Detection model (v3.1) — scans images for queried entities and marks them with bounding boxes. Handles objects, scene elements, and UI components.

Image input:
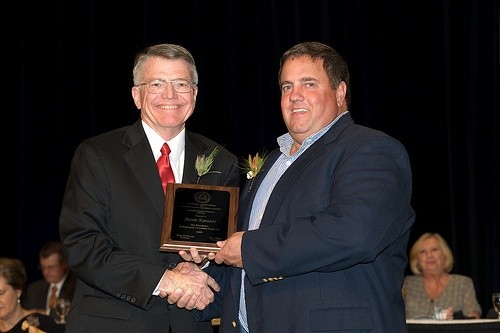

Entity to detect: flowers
[232,151,272,193]
[194,145,223,184]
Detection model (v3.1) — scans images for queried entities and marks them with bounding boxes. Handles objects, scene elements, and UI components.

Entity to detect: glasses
[135,78,196,93]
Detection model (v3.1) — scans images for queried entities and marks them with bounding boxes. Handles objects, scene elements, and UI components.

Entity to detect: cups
[433,298,450,321]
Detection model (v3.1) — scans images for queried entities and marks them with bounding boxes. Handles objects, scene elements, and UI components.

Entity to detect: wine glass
[55,299,70,324]
[492,294,500,321]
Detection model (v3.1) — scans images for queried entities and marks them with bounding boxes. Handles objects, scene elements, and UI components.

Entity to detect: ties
[49,286,57,309]
[156,143,176,196]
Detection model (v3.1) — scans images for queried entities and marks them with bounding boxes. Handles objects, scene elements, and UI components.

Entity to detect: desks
[406,317,500,333]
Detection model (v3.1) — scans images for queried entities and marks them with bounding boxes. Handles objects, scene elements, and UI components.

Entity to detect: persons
[58,43,243,333]
[0,258,61,333]
[157,41,416,333]
[22,241,77,333]
[401,232,482,320]
